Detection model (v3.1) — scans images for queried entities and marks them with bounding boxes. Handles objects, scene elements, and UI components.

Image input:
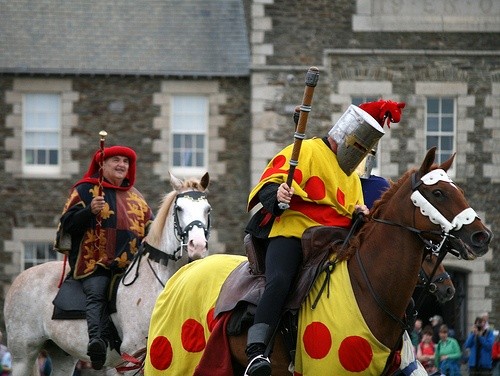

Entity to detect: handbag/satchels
[439,359,462,376]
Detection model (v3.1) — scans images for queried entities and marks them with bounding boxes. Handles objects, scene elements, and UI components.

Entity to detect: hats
[429,315,443,326]
[475,313,488,326]
[73,145,136,191]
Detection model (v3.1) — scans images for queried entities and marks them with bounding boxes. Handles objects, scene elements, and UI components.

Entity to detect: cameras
[474,317,483,330]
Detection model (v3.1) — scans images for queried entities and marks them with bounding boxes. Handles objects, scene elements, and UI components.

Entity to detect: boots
[81,276,110,371]
[245,323,273,376]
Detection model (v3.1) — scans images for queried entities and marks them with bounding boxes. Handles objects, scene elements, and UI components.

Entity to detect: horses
[143,146,493,376]
[4,171,212,376]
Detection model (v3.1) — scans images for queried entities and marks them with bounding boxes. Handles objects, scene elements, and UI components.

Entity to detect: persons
[244,99,405,376]
[409,310,500,376]
[0,330,51,376]
[53,135,155,371]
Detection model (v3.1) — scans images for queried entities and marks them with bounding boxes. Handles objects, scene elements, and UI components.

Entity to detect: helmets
[328,104,386,180]
